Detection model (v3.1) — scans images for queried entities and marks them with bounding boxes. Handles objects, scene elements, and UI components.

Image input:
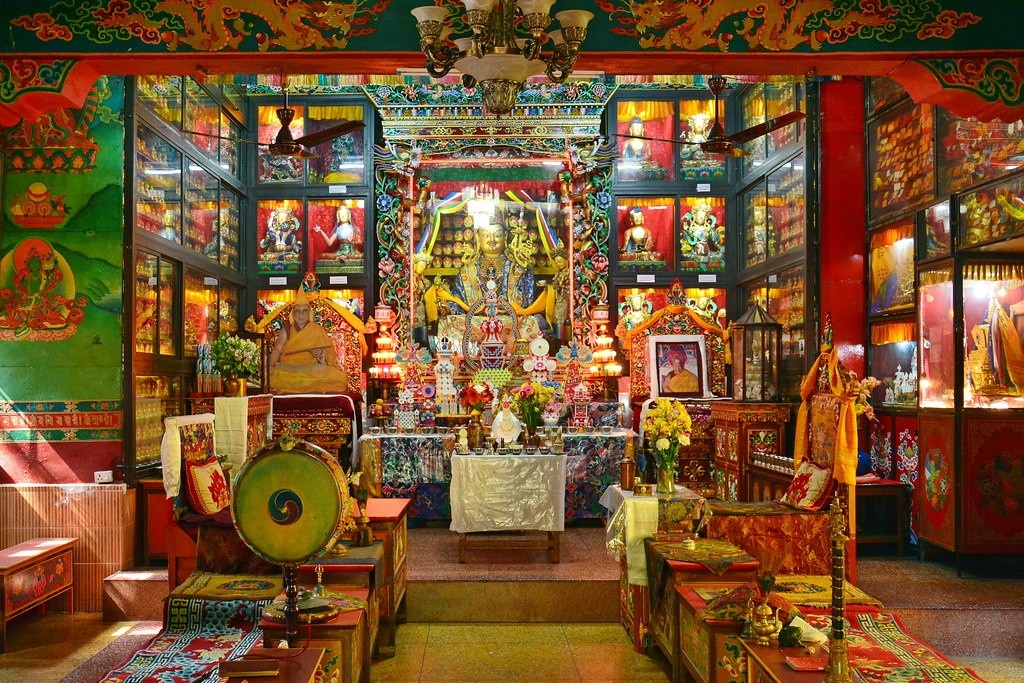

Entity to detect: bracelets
[320,353,325,357]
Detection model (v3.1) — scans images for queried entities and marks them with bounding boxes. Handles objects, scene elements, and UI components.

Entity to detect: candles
[369,325,401,378]
[589,325,622,376]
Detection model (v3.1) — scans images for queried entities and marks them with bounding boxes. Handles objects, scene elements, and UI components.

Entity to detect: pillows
[774,454,835,513]
[184,455,231,515]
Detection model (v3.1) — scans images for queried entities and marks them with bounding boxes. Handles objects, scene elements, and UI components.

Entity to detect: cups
[473,448,484,454]
[539,447,550,454]
[524,448,537,454]
[454,447,459,453]
[497,448,509,454]
[511,448,522,455]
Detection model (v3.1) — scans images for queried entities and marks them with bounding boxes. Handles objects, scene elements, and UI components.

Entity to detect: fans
[611,75,808,158]
[180,72,366,158]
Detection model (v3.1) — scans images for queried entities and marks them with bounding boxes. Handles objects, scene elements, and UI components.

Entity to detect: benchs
[856,476,910,556]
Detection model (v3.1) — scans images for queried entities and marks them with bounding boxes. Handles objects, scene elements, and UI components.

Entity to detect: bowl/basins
[633,483,652,495]
[368,427,468,435]
[535,426,615,434]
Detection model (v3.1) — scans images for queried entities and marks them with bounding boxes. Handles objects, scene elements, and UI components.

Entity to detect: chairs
[703,313,860,585]
[160,412,283,577]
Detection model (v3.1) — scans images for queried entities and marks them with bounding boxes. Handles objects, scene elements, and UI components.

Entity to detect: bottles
[683,536,695,551]
[621,456,635,489]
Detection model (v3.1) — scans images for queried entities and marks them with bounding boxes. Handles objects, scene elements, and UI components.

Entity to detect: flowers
[458,380,555,428]
[212,332,262,378]
[644,396,692,492]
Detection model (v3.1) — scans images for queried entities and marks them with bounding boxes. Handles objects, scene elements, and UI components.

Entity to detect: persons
[662,344,698,392]
[260,206,304,253]
[618,205,662,261]
[680,111,713,161]
[314,204,365,259]
[436,202,556,357]
[681,200,723,261]
[618,116,661,168]
[269,280,348,393]
[619,292,650,330]
[685,288,717,317]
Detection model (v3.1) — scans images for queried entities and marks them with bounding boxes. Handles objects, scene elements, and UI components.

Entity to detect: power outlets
[94,470,113,483]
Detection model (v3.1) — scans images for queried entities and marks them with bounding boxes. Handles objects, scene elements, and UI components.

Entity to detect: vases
[467,416,540,450]
[222,374,240,397]
[656,462,675,493]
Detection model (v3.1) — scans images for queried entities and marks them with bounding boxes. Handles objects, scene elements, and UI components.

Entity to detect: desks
[709,401,793,503]
[359,428,639,519]
[450,447,567,562]
[599,484,865,683]
[272,394,365,497]
[139,464,233,566]
[0,537,79,654]
[631,395,732,488]
[175,498,413,683]
[214,394,274,489]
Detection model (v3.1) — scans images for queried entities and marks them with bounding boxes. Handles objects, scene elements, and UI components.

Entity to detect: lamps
[411,0,594,120]
